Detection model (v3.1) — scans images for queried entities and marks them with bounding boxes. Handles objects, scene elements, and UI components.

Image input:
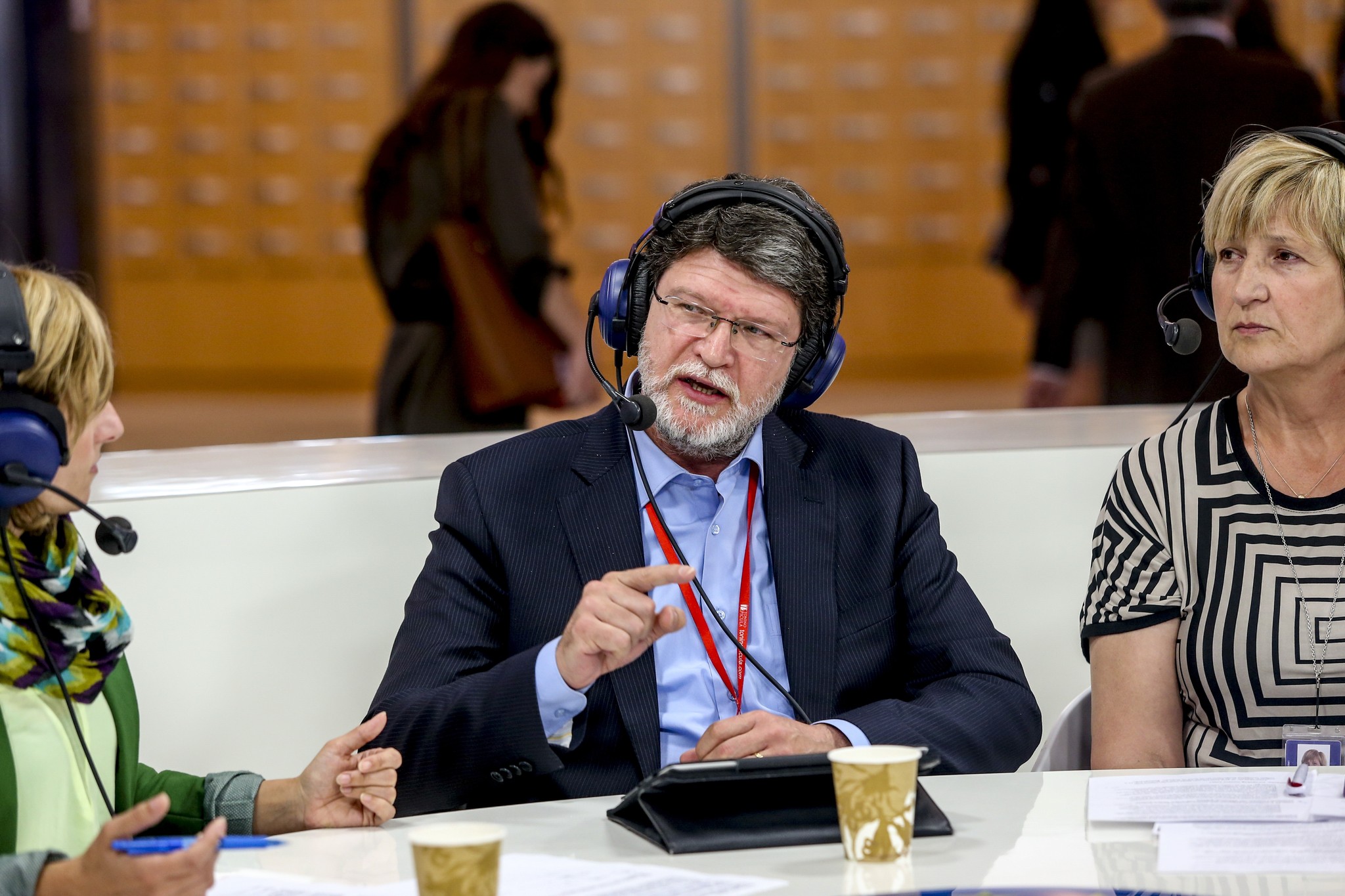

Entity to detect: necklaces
[1244,392,1345,499]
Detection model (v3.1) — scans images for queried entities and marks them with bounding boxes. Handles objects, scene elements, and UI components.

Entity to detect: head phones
[0,269,66,505]
[593,181,849,409]
[1190,126,1344,321]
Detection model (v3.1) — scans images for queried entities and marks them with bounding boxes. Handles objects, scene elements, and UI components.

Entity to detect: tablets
[615,742,941,818]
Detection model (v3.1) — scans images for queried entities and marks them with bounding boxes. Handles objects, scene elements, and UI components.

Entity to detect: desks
[203,762,1345,894]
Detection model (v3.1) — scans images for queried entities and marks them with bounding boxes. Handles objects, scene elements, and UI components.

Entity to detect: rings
[752,753,765,759]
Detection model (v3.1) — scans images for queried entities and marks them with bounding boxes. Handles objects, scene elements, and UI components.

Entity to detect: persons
[358,0,601,437]
[0,258,405,896]
[1301,750,1327,767]
[986,0,1118,409]
[353,169,1043,821]
[1076,126,1345,770]
[1021,1,1327,417]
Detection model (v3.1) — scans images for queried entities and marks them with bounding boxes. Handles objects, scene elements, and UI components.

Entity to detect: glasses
[653,281,810,362]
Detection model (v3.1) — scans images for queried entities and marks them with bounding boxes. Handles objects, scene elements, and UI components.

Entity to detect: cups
[828,745,922,863]
[412,821,506,895]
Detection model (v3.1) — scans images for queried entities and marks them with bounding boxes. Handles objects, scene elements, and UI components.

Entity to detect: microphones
[5,462,137,554]
[1156,279,1201,355]
[583,294,657,430]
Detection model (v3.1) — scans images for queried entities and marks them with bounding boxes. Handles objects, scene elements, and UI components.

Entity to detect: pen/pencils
[1286,764,1308,795]
[115,836,285,857]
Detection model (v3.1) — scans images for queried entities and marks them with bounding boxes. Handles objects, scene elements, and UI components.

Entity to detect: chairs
[1031,684,1093,772]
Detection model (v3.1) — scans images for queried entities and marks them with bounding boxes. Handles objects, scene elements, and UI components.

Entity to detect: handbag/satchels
[426,220,572,409]
